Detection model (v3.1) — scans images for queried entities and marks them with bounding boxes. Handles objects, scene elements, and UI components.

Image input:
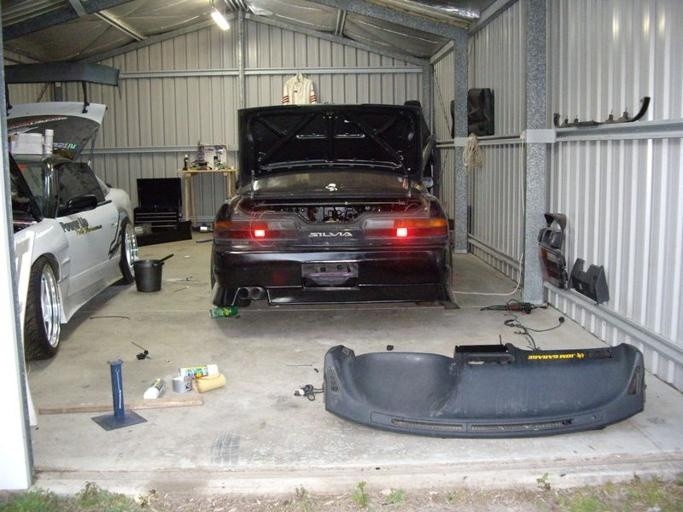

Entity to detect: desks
[180,168,237,221]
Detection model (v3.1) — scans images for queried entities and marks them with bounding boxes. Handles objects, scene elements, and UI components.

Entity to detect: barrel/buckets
[133,259,164,292]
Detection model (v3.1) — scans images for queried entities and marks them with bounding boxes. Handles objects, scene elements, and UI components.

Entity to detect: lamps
[209,0,231,32]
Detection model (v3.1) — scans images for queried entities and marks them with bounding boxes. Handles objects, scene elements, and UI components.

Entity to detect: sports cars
[209,102,454,307]
[8,101,140,362]
